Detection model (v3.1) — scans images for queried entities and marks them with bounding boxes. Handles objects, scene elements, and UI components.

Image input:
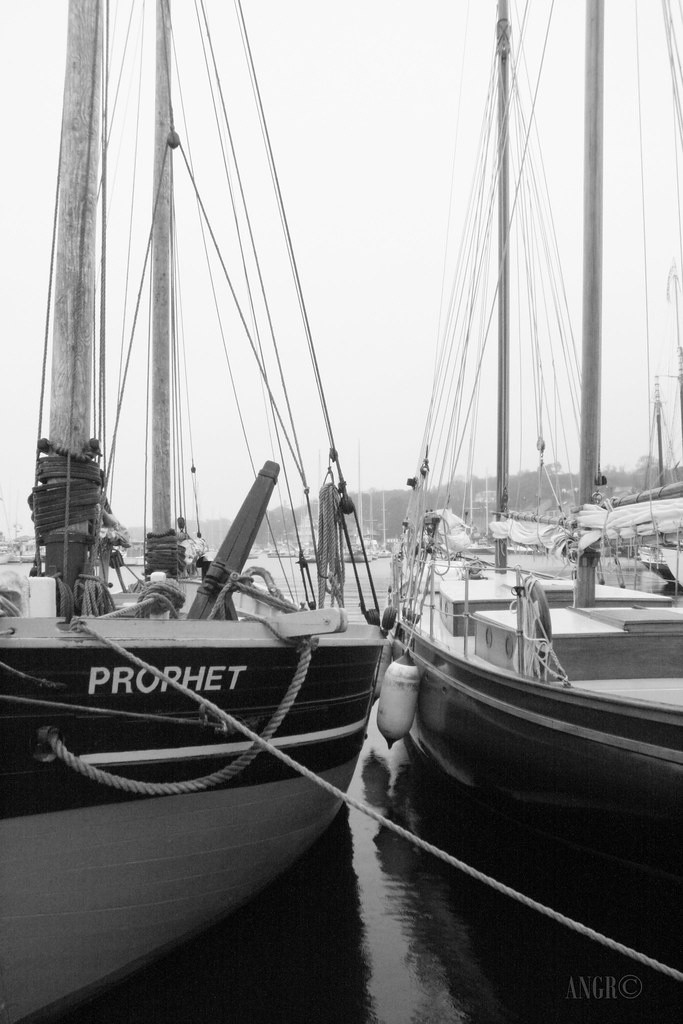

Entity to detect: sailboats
[1,426,681,573]
[376,1,682,885]
[1,0,403,1024]
